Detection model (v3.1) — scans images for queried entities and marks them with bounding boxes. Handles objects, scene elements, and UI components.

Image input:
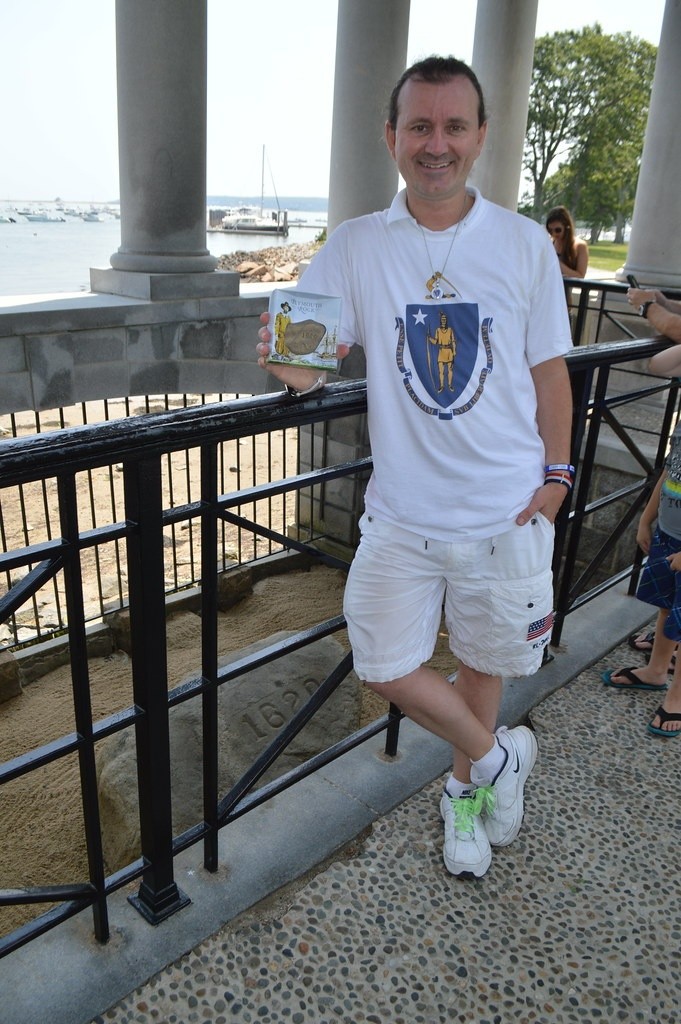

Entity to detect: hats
[281,301,291,311]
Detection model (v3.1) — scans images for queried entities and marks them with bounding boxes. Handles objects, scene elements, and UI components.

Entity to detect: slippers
[602,666,667,690]
[646,704,681,737]
[628,631,678,675]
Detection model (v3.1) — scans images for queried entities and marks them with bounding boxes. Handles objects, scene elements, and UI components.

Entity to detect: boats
[206,143,328,237]
[0,191,120,226]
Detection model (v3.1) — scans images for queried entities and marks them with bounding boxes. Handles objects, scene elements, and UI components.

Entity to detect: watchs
[638,300,656,319]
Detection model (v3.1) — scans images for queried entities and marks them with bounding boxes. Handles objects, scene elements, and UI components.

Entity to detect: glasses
[547,226,569,233]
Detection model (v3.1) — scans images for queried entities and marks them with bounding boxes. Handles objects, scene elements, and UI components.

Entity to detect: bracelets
[544,463,575,473]
[544,473,573,489]
[285,368,327,399]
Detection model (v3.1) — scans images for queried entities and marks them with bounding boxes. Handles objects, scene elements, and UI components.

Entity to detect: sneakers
[469,726,538,848]
[439,785,491,878]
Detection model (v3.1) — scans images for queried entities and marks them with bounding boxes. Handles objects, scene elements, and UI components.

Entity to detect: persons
[545,206,589,340]
[273,302,292,361]
[628,340,681,676]
[256,59,580,882]
[601,411,681,738]
[624,273,681,345]
[428,311,457,393]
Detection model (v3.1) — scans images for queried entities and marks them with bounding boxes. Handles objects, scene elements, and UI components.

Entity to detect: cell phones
[627,274,639,288]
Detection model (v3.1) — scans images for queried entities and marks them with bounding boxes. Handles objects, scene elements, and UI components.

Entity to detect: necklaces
[407,191,467,300]
